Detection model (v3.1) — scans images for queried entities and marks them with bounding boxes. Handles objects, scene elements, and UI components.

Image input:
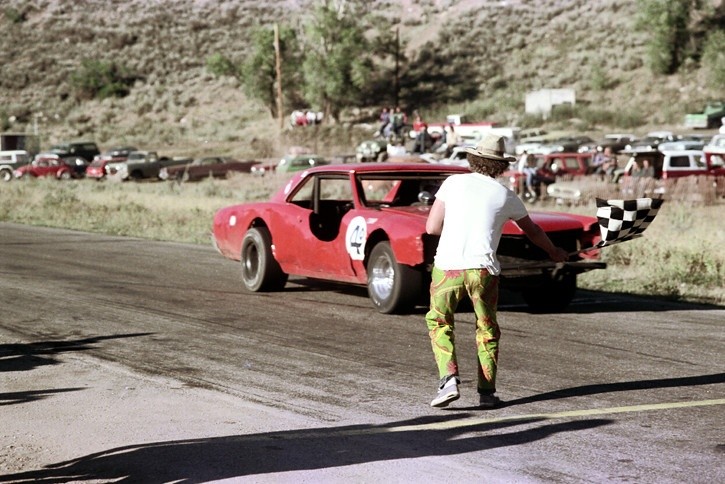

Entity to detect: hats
[464,132,516,162]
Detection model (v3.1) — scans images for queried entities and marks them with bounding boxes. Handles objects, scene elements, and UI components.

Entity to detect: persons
[519,143,616,201]
[425,133,568,406]
[291,107,437,153]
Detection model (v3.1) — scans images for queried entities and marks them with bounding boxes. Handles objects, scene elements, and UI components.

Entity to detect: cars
[275,152,331,174]
[157,154,262,184]
[249,159,281,176]
[0,141,195,181]
[419,111,725,209]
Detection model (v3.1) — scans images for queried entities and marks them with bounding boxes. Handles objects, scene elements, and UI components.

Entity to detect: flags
[595,197,664,249]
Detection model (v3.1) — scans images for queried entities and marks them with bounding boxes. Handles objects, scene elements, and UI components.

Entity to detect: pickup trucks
[682,101,725,129]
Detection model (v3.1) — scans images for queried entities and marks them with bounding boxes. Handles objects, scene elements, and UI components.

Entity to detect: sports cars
[211,162,609,316]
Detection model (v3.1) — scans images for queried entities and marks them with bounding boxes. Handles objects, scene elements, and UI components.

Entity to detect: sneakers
[430,384,460,407]
[480,395,500,406]
